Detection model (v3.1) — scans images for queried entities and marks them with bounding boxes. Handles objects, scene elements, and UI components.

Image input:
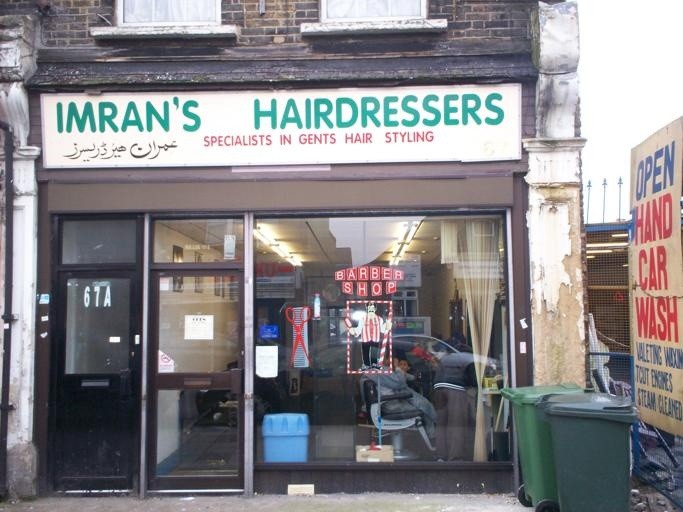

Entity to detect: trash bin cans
[499,381,638,512]
[262,412,310,463]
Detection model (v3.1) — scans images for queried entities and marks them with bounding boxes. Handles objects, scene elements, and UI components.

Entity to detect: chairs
[362,375,438,452]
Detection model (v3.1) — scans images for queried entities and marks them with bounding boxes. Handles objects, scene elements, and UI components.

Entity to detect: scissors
[285,306,312,368]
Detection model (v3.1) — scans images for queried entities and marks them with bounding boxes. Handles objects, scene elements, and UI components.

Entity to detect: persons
[371,348,438,440]
[349,301,392,371]
[432,341,502,463]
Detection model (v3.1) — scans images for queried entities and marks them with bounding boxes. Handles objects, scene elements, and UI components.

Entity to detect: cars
[280,334,498,420]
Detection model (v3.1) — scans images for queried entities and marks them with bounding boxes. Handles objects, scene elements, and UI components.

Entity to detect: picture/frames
[171,245,227,297]
